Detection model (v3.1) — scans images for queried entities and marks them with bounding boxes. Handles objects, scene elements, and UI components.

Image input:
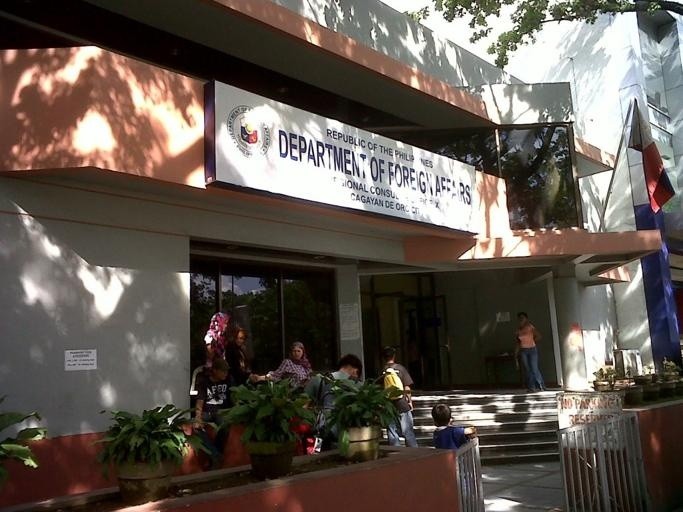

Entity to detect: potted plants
[315,370,412,462]
[218,375,316,478]
[90,403,218,503]
[592,356,683,406]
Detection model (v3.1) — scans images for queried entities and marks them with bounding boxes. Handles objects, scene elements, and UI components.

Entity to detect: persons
[190,311,362,455]
[432,404,477,451]
[513,312,546,394]
[376,346,420,449]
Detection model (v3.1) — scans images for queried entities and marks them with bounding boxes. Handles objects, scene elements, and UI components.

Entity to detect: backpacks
[381,364,403,400]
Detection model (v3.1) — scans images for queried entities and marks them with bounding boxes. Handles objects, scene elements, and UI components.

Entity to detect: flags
[626,99,676,217]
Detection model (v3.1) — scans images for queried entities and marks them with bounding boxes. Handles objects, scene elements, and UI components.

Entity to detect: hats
[214,358,230,370]
